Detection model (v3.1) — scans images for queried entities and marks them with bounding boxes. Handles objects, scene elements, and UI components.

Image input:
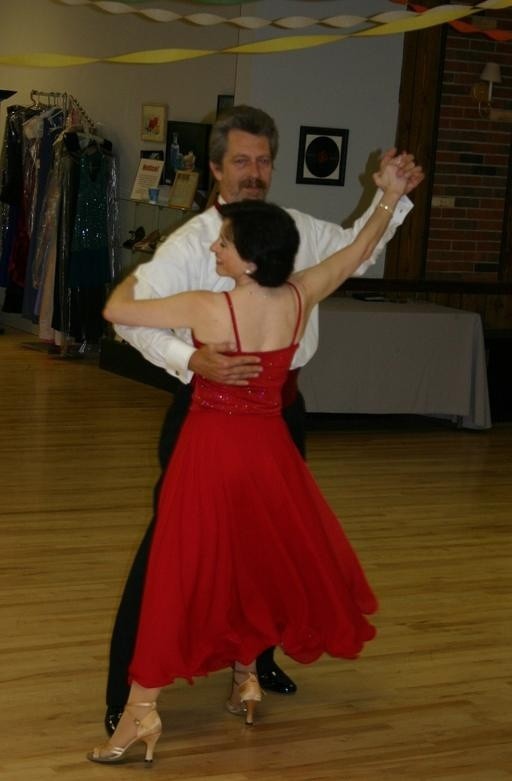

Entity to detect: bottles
[174,152,183,173]
[170,131,179,168]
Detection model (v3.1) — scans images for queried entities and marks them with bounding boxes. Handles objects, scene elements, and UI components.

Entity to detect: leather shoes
[257,657,297,695]
[104,701,126,737]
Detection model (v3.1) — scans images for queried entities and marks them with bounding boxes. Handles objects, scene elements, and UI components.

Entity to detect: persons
[103,103,427,740]
[86,155,414,766]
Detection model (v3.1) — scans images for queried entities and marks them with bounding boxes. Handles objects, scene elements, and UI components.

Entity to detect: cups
[148,187,160,205]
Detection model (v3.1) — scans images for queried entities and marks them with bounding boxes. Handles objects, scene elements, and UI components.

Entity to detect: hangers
[11,89,103,147]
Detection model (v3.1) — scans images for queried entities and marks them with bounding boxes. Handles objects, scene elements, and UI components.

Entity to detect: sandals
[122,225,170,253]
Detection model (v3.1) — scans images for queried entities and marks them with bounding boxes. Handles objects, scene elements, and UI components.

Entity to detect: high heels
[224,670,262,727]
[85,700,163,764]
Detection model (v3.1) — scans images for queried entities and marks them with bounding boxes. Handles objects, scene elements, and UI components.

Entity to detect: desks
[295,296,494,432]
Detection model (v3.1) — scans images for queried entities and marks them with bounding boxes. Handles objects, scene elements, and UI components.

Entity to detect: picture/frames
[216,93,235,120]
[295,125,349,187]
[166,169,200,209]
[141,103,169,144]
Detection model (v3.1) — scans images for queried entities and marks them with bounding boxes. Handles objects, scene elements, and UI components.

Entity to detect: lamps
[470,61,502,119]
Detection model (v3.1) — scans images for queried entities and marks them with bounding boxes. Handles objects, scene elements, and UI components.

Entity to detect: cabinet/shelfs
[118,198,202,255]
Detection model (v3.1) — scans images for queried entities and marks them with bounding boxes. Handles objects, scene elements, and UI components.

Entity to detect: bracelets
[376,202,394,216]
